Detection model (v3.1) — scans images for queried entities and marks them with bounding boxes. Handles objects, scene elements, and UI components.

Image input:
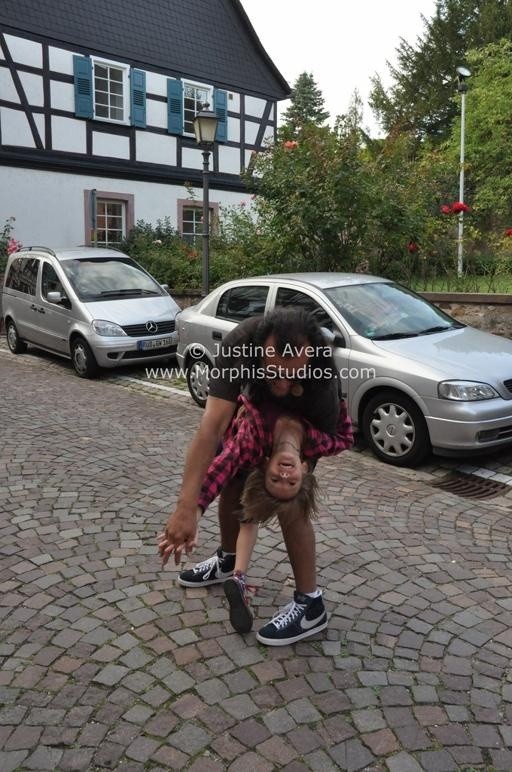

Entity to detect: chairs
[224,294,253,321]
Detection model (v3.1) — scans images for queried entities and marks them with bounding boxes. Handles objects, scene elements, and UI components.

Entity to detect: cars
[173,271,512,465]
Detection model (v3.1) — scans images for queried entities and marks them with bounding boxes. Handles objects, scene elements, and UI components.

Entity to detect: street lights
[192,101,222,299]
[453,65,472,279]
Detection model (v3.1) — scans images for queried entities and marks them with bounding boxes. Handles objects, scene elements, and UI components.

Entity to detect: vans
[2,244,184,380]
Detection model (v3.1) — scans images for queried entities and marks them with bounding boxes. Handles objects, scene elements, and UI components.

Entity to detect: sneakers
[223,571,254,633]
[177,546,236,588]
[255,590,328,648]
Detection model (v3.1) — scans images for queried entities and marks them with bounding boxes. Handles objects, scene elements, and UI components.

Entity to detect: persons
[159,306,344,646]
[156,390,354,633]
